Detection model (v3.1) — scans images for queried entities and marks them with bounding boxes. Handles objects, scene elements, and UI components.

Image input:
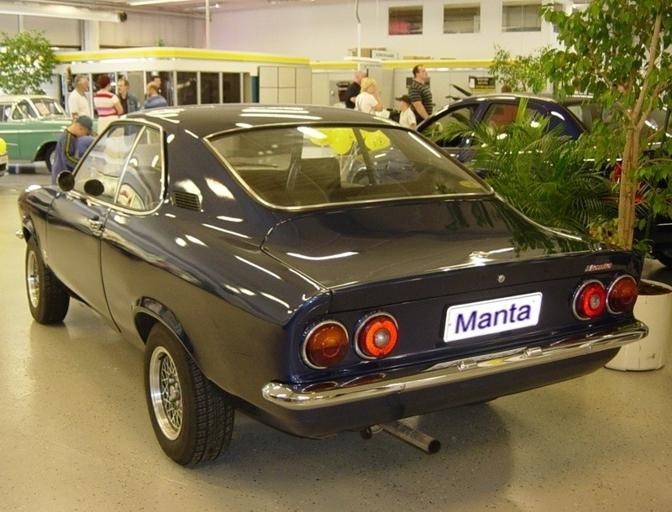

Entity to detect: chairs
[286,157,342,191]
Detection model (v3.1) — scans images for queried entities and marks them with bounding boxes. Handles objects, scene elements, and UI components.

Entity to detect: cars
[0,137,9,177]
[16,102,650,469]
[0,95,98,172]
[346,93,672,269]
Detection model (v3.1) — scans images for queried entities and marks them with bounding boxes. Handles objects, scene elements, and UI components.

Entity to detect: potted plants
[488,0,672,372]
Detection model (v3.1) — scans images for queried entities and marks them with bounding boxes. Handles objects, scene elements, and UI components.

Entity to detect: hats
[78,116,92,128]
[361,79,374,91]
[396,96,410,102]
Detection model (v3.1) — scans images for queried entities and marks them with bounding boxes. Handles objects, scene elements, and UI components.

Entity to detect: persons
[354,77,384,115]
[50,114,92,185]
[408,63,433,125]
[346,70,364,110]
[68,74,168,143]
[395,94,417,130]
